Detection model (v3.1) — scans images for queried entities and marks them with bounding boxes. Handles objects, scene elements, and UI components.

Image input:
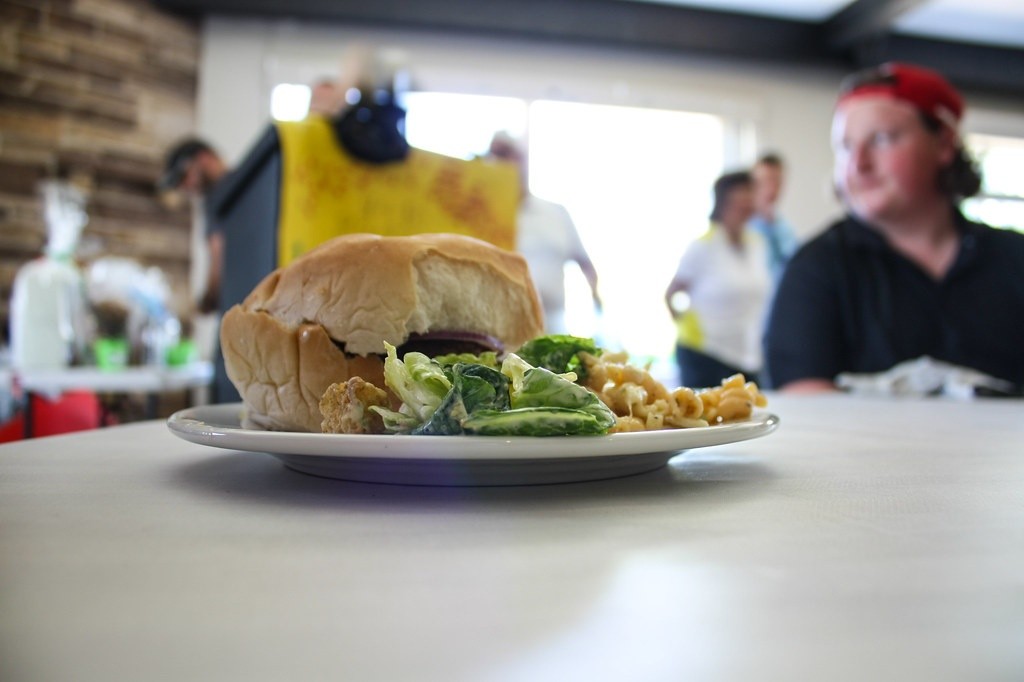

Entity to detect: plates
[166,403,779,488]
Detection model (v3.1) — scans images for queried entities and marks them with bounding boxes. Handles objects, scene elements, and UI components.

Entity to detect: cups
[94,339,129,368]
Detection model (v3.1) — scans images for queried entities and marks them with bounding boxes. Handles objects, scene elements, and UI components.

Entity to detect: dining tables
[0,397,1024,682]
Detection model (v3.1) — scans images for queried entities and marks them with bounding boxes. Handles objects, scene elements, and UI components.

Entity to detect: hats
[832,60,967,136]
[708,172,751,221]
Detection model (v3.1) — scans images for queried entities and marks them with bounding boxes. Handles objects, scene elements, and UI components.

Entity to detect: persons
[752,156,801,389]
[482,134,605,335]
[667,173,773,386]
[766,61,1024,403]
[160,134,244,403]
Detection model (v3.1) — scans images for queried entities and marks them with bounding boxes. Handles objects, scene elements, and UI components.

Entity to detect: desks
[20,366,213,418]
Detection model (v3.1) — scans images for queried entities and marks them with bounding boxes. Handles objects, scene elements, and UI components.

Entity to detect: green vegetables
[365,332,618,437]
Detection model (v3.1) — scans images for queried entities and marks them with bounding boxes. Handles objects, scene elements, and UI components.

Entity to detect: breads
[218,234,543,429]
